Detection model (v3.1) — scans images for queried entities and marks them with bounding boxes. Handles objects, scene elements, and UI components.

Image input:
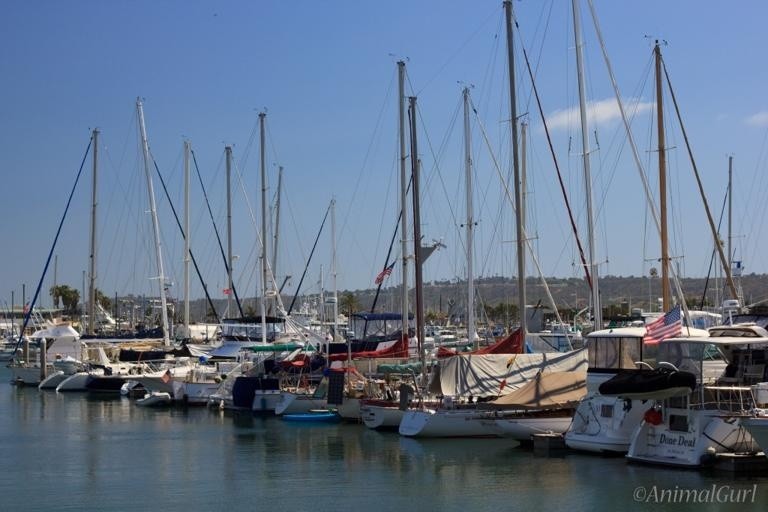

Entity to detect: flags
[375,262,395,285]
[643,303,682,345]
